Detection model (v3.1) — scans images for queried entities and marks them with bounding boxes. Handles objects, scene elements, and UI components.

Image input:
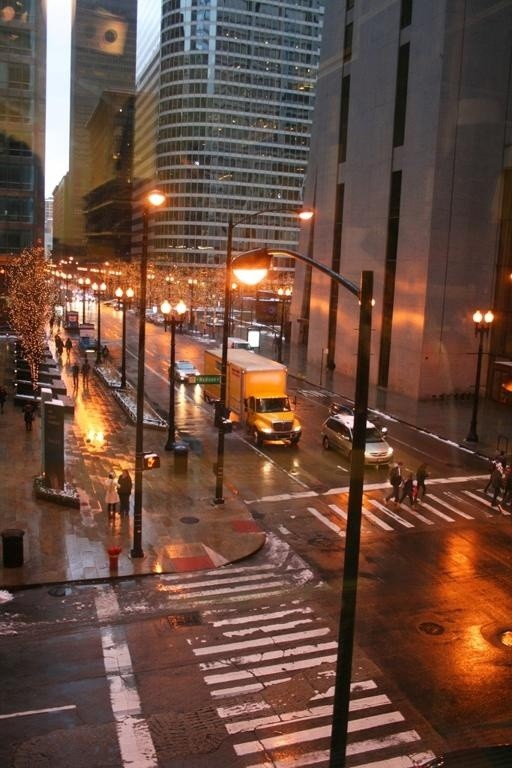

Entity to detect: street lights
[214,205,317,505]
[161,298,187,449]
[43,257,291,387]
[132,188,165,559]
[466,309,494,444]
[232,246,374,768]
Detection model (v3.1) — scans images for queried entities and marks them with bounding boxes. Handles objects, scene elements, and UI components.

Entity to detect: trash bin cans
[174,445,188,474]
[0,529,26,568]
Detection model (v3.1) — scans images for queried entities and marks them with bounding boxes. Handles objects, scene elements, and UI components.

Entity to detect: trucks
[202,347,302,447]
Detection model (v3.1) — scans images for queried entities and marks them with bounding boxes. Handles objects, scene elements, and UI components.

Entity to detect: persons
[483,448,511,507]
[80,359,91,386]
[103,472,121,519]
[117,468,133,514]
[101,344,110,364]
[65,337,73,355]
[72,362,80,388]
[1,384,9,414]
[399,470,415,505]
[413,461,429,503]
[21,404,35,432]
[382,464,401,505]
[49,315,64,357]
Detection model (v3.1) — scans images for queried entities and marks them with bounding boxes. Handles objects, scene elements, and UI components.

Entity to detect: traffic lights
[142,453,160,469]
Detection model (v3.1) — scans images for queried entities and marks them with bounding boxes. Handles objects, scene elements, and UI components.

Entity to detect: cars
[321,401,394,466]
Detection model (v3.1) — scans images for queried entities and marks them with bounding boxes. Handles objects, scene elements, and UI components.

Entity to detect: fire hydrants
[108,545,122,571]
